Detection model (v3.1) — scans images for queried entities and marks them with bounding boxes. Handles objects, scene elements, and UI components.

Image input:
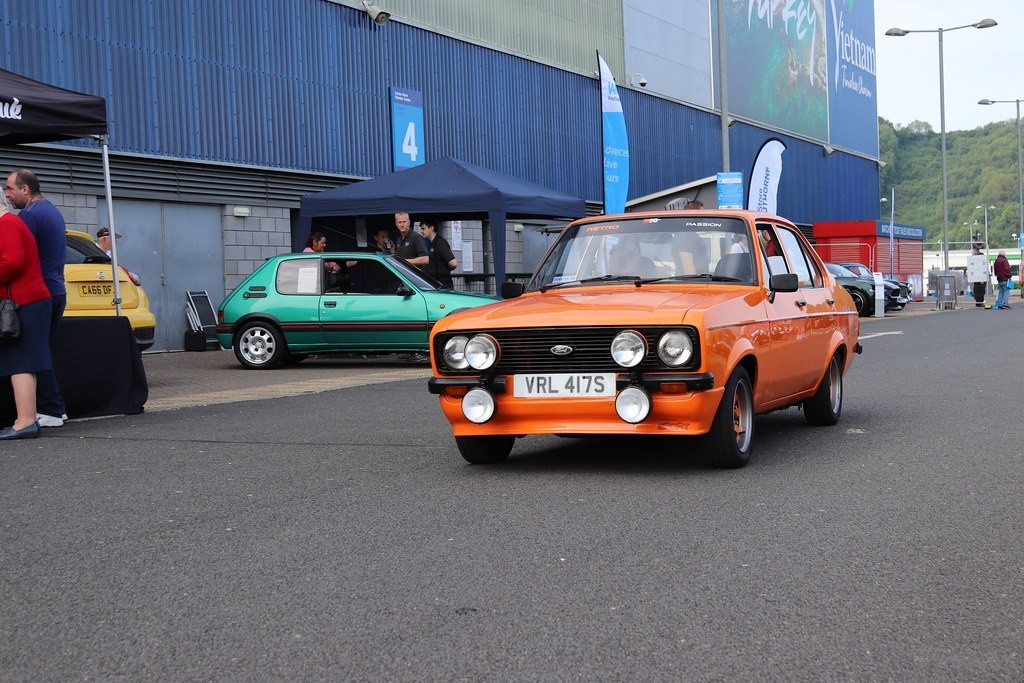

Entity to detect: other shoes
[998,305,1006,309]
[976,304,984,307]
[0,420,40,440]
[1003,304,1010,308]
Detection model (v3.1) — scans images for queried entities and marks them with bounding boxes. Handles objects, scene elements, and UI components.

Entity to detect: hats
[97,228,121,239]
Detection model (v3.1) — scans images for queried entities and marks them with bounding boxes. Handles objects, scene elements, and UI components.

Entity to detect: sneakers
[14,414,63,426]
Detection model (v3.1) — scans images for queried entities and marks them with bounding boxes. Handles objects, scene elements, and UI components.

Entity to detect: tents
[298,156,585,295]
[0,67,122,315]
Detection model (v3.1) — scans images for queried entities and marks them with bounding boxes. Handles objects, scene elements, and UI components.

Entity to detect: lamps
[880,198,888,202]
[630,73,647,88]
[361,0,390,25]
[233,208,250,216]
[514,225,524,231]
[823,145,835,156]
[718,114,735,126]
[876,160,888,168]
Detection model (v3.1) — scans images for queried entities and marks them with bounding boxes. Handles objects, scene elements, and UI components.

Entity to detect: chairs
[327,274,352,294]
[712,252,752,284]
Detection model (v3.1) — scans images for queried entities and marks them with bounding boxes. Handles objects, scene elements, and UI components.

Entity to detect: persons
[994,250,1012,309]
[670,200,709,274]
[973,253,985,307]
[731,234,749,254]
[368,230,392,253]
[5,168,68,428]
[86,227,121,263]
[0,185,53,441]
[761,229,777,257]
[388,210,430,272]
[420,219,458,290]
[303,232,340,274]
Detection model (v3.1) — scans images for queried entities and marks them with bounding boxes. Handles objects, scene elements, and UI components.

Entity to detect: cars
[990,258,1022,289]
[62,229,156,352]
[430,208,865,469]
[834,262,914,312]
[217,251,506,371]
[824,262,888,317]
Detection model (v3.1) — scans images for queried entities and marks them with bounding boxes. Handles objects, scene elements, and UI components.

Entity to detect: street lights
[976,206,996,255]
[978,98,1023,299]
[884,18,998,273]
[963,222,979,255]
